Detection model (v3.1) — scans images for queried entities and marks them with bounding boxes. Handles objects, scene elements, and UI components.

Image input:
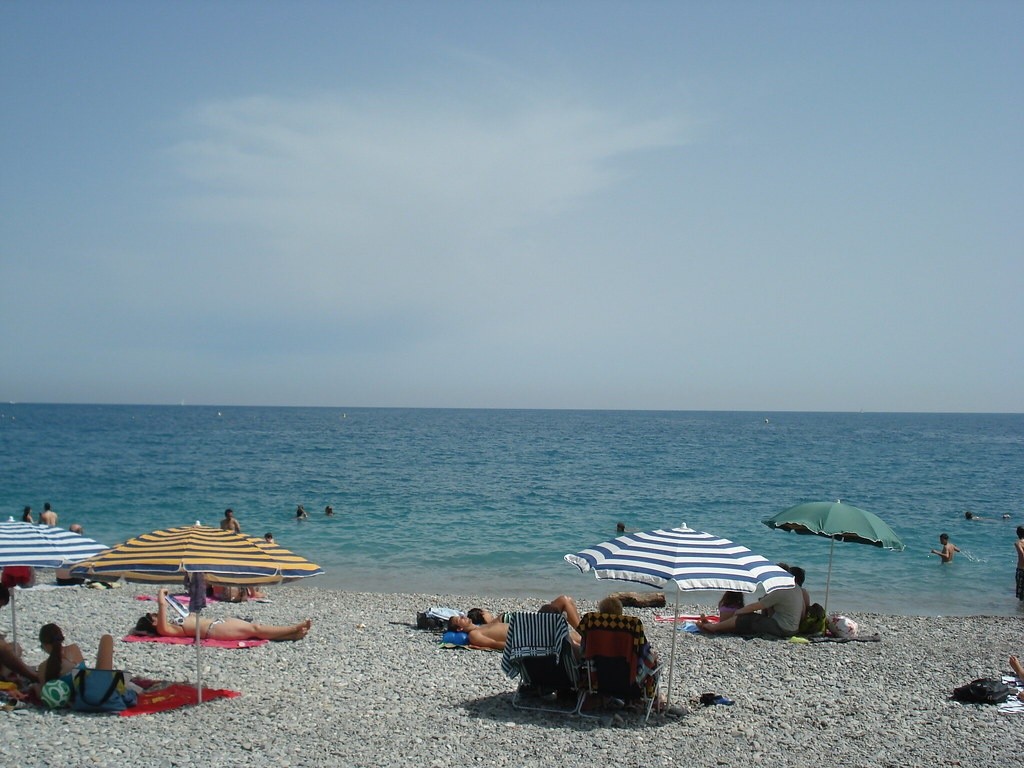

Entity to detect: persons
[22,507,34,522]
[931,534,961,564]
[615,523,628,533]
[324,506,334,517]
[69,524,82,534]
[1009,655,1024,702]
[264,533,274,543]
[1014,526,1024,601]
[296,506,307,520]
[965,512,1010,523]
[0,583,39,684]
[37,623,113,693]
[448,596,581,649]
[696,564,810,638]
[39,504,57,526]
[220,509,240,533]
[598,597,666,706]
[136,589,312,641]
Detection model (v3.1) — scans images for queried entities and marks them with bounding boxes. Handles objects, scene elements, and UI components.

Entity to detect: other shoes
[700,693,735,706]
[696,621,714,634]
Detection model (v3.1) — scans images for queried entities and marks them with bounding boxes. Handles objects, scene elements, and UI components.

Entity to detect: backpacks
[953,679,1009,704]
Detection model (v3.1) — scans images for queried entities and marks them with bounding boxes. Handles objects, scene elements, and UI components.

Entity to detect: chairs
[577,611,665,724]
[500,610,584,715]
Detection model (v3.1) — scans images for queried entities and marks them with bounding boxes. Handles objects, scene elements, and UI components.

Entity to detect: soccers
[41,679,70,709]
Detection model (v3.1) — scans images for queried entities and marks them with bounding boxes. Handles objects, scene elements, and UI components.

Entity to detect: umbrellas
[70,521,325,705]
[562,522,795,715]
[0,517,110,654]
[762,499,906,611]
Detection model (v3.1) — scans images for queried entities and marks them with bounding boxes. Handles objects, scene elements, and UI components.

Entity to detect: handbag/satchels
[799,603,858,638]
[71,667,132,711]
